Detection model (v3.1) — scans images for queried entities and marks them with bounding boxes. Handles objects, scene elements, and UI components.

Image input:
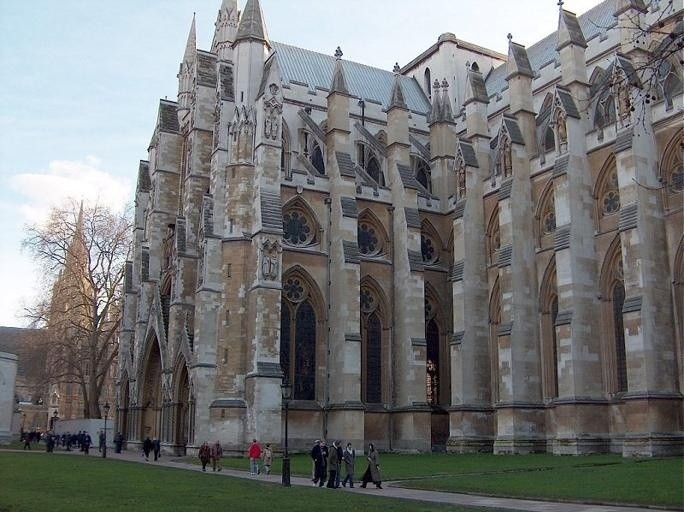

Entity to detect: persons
[311,438,384,489]
[261,442,273,474]
[211,440,223,472]
[21,430,123,456]
[143,437,151,462]
[152,439,161,461]
[197,440,210,471]
[248,438,261,475]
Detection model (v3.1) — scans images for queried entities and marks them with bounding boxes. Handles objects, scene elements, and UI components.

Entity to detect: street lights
[53,409,59,436]
[279,378,294,486]
[102,403,110,458]
